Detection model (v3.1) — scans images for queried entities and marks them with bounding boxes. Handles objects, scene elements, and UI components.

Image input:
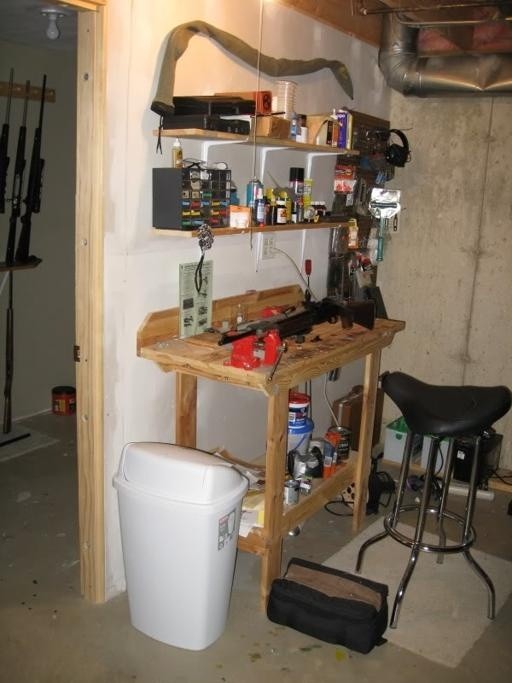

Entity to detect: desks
[137,285,405,612]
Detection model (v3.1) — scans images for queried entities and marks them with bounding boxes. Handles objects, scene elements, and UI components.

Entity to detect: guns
[217,295,376,347]
[0,67,47,267]
[2,270,13,436]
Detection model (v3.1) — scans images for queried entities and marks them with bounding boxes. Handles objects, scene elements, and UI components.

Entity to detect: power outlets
[261,234,276,259]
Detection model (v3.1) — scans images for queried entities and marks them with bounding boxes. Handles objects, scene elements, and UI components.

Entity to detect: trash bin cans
[112,441,249,651]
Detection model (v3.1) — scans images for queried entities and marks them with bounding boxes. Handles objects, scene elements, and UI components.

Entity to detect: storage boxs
[384,416,449,474]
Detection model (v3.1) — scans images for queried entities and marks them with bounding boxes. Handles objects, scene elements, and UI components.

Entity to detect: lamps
[41,9,67,40]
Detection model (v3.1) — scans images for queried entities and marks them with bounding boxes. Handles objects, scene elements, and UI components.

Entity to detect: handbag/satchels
[268,557,388,654]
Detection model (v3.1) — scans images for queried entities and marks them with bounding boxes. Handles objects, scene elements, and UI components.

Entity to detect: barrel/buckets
[287,421,314,456]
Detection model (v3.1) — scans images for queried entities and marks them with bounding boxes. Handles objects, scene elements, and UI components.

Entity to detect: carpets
[320,515,512,671]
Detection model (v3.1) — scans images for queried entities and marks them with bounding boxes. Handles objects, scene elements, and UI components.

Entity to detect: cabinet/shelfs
[153,128,360,238]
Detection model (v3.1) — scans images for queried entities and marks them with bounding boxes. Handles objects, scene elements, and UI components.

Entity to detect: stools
[356,372,512,628]
